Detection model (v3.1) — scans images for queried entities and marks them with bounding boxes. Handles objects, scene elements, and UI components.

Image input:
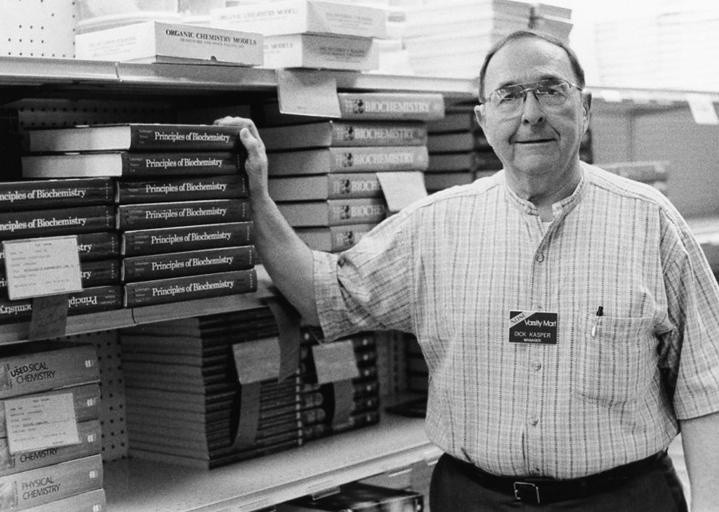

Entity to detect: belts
[442,455,670,505]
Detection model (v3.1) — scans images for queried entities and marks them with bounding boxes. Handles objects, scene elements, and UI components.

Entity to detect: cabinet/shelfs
[0,55,719,512]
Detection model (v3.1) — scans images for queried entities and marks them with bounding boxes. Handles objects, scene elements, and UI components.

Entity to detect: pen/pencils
[591,306,603,337]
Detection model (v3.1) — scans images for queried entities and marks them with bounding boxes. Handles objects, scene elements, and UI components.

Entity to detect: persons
[207,28,719,510]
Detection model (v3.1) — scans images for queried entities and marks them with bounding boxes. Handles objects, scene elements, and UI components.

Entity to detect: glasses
[486,79,582,115]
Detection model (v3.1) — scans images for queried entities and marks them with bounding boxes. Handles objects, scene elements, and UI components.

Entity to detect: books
[579,130,669,197]
